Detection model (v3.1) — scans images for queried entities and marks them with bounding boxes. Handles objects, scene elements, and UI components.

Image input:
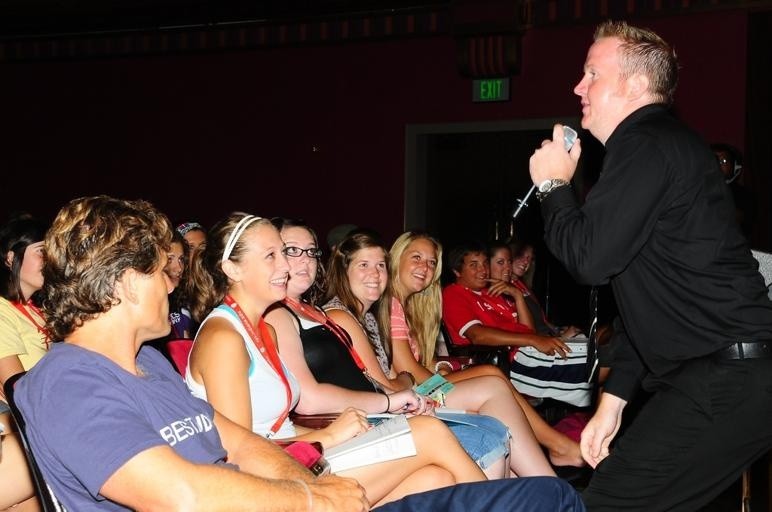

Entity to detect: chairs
[3,370,68,511]
[439,319,555,423]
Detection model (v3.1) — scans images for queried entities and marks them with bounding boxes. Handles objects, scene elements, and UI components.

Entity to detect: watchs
[534,177,572,200]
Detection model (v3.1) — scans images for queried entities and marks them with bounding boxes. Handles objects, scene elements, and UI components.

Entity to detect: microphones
[513,125,577,221]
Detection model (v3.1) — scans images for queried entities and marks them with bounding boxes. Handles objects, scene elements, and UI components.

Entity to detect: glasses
[286,247,324,258]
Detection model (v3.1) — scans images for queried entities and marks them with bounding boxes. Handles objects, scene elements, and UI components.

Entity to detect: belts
[714,342,771,359]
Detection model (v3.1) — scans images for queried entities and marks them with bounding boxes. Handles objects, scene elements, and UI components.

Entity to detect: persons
[527,20,771,512]
[1,195,615,512]
